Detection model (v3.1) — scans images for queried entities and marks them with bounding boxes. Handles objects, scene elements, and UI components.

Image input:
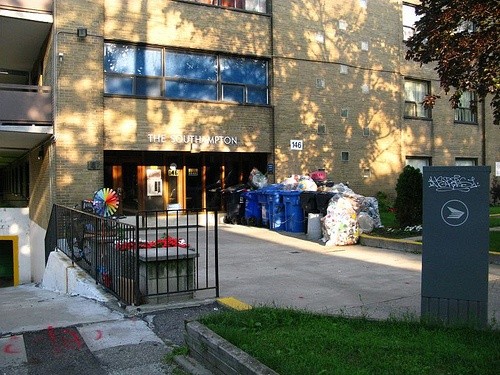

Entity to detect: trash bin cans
[222,185,338,235]
